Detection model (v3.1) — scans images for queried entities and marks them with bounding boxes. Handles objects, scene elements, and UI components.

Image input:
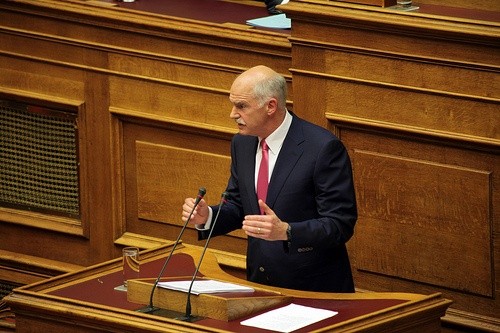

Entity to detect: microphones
[173,191,230,323]
[135,188,206,313]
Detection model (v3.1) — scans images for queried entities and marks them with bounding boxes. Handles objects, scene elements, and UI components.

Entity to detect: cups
[122,246,141,285]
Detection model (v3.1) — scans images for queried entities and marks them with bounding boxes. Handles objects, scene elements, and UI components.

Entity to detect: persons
[181,65,358,293]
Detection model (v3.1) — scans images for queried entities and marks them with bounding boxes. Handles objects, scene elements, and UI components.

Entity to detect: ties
[257,138,270,215]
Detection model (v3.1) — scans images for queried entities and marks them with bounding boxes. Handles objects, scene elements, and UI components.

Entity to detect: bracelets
[286,225,293,241]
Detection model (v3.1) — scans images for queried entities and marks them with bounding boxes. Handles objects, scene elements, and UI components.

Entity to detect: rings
[258,227,261,233]
